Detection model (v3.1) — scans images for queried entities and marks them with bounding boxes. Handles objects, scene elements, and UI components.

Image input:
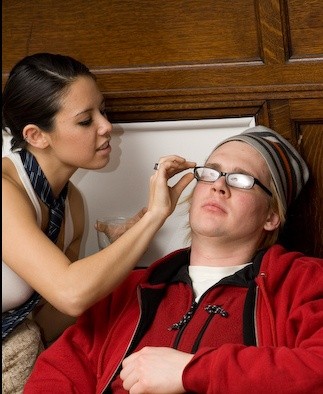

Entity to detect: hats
[204,124,309,211]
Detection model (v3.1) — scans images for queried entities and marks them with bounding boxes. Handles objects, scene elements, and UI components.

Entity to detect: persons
[23,125,322,393]
[2,52,196,342]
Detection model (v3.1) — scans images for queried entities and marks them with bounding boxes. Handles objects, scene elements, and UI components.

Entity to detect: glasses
[193,166,272,198]
